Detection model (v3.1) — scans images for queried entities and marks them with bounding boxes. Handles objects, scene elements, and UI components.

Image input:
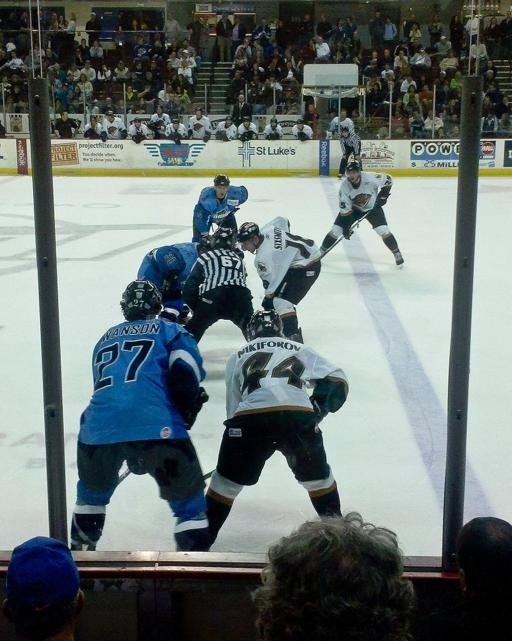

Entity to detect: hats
[88,104,306,125]
[5,535,83,614]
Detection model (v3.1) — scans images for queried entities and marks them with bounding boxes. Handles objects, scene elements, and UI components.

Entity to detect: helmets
[213,173,230,189]
[195,221,261,249]
[343,157,362,177]
[242,307,285,340]
[341,125,350,132]
[118,277,164,320]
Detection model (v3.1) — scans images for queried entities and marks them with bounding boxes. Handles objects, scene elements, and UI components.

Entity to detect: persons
[1,537,85,641]
[237,216,321,344]
[252,513,419,641]
[191,174,248,243]
[408,518,512,641]
[1,7,511,177]
[319,160,404,266]
[183,227,254,344]
[70,280,210,551]
[137,242,199,326]
[204,309,349,552]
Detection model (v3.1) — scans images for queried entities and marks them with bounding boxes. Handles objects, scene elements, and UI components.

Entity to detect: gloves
[341,220,355,241]
[375,187,392,208]
[83,128,309,141]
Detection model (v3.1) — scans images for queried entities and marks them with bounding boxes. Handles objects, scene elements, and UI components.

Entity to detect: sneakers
[336,172,344,178]
[393,249,403,265]
